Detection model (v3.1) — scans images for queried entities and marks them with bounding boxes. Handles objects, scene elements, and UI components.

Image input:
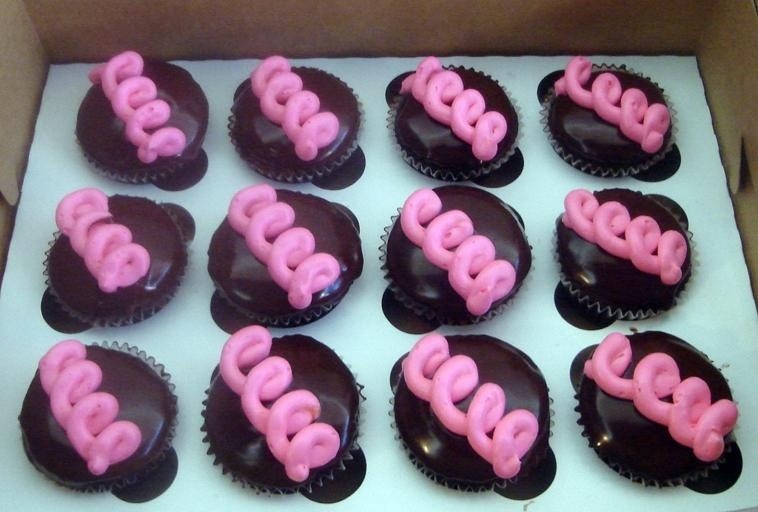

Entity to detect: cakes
[389,331,557,500]
[207,183,364,334]
[204,324,366,504]
[381,184,533,334]
[537,56,681,182]
[75,48,207,190]
[234,56,367,190]
[17,339,179,502]
[570,328,743,494]
[41,187,195,333]
[553,187,692,331]
[385,57,524,188]
